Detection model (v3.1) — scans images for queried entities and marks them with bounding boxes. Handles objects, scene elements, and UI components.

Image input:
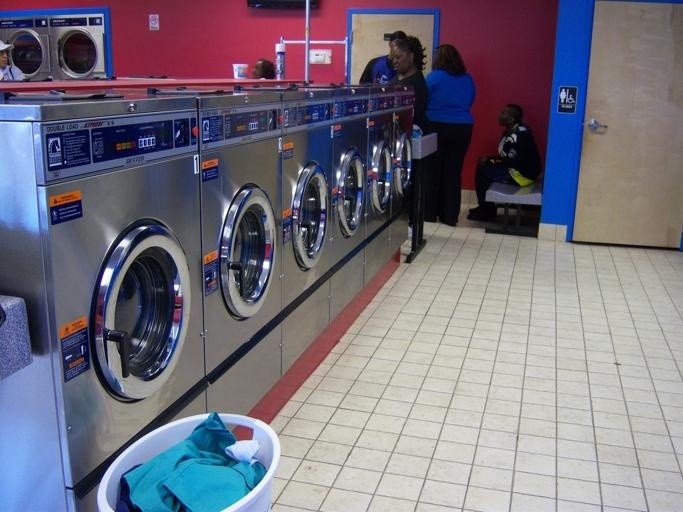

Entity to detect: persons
[424,42,476,227]
[465,103,541,221]
[0,40,25,83]
[386,35,429,227]
[358,30,408,84]
[252,58,275,79]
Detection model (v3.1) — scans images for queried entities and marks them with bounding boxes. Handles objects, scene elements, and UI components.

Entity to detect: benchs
[482,180,543,237]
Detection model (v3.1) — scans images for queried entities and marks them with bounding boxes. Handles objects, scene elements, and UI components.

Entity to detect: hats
[0,39,14,51]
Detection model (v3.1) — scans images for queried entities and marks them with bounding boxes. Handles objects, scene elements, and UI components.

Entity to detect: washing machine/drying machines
[50,14,110,81]
[0,88,415,474]
[1,13,49,81]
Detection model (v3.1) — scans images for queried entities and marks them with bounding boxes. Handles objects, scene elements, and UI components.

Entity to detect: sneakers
[467,206,497,223]
[422,216,456,227]
